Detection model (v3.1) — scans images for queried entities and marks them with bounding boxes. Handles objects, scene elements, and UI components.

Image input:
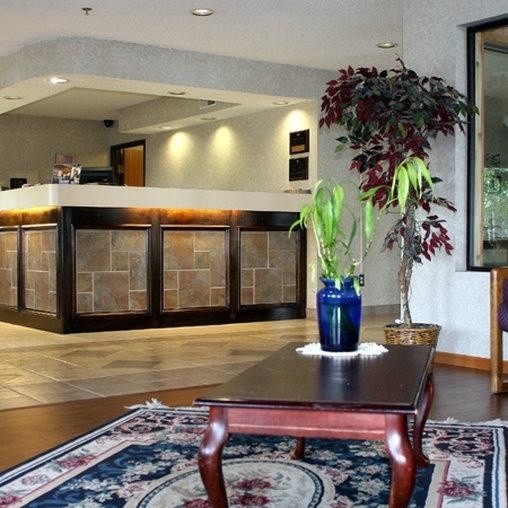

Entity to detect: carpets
[0,401,508,508]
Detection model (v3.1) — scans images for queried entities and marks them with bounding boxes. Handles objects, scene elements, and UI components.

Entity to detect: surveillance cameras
[104,120,113,127]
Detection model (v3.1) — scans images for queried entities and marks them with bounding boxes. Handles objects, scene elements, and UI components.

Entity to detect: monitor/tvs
[79,167,114,185]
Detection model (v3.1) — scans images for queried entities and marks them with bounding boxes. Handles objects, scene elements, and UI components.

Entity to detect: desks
[192,341,438,507]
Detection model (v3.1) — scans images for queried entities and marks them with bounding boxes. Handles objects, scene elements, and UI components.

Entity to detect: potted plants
[285,155,430,350]
[319,50,479,347]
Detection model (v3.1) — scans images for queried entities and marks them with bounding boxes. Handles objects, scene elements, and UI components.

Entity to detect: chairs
[487,265,507,394]
